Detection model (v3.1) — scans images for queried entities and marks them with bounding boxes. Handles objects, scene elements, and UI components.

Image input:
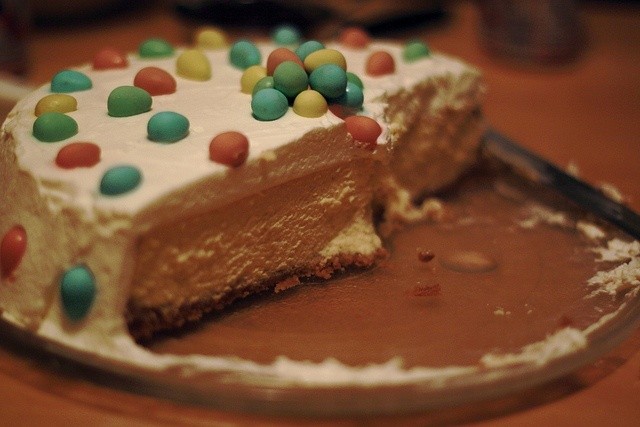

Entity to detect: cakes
[0,27,486,381]
